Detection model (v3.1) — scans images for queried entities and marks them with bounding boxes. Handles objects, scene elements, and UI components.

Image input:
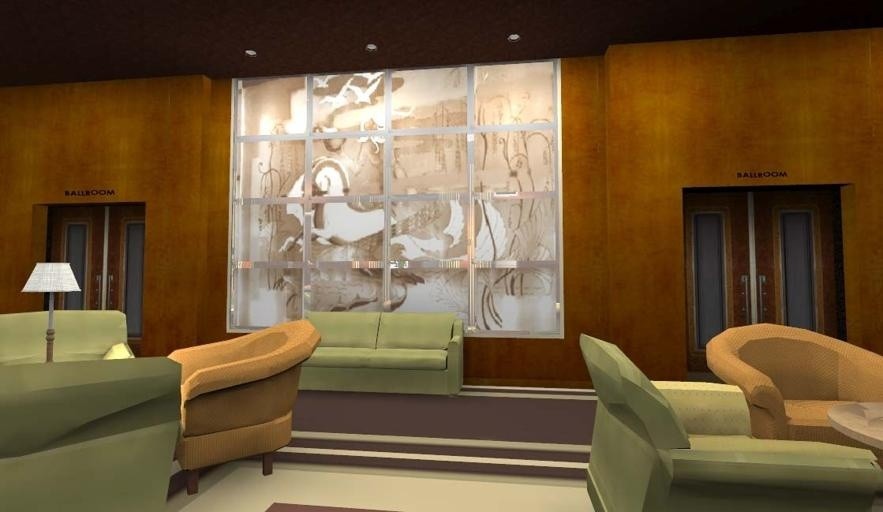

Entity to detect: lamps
[20,260,82,361]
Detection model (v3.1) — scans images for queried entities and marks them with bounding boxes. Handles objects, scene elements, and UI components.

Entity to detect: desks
[824,400,883,451]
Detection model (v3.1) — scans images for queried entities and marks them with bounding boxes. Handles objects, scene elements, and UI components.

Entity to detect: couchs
[165,317,323,495]
[0,353,185,511]
[0,310,135,364]
[296,310,467,396]
[574,330,883,510]
[705,319,883,466]
